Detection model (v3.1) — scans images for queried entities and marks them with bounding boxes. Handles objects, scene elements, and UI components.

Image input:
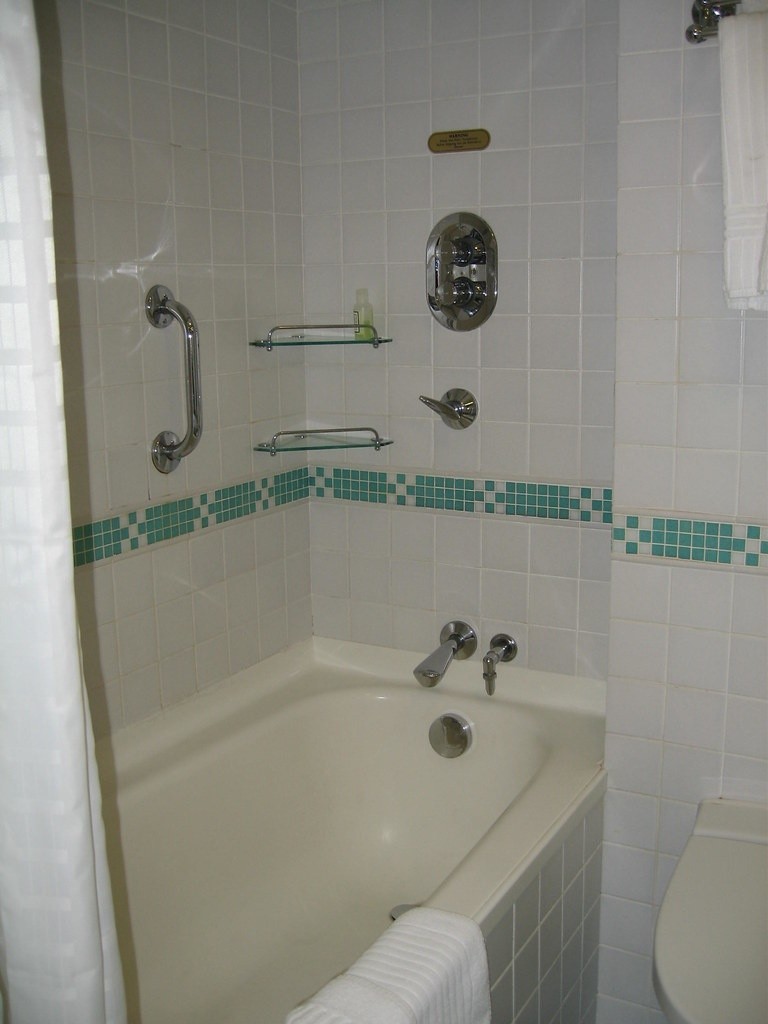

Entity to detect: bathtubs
[97,633,605,1022]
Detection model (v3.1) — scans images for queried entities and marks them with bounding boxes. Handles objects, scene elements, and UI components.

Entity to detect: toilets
[649,795,768,1023]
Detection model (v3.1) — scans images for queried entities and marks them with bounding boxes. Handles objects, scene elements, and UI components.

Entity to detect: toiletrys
[349,286,376,344]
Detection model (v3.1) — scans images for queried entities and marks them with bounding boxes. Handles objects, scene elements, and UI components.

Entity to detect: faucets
[411,618,479,689]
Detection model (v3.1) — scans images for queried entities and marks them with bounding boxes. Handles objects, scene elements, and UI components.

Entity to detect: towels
[284,903,493,1024]
[713,9,767,313]
